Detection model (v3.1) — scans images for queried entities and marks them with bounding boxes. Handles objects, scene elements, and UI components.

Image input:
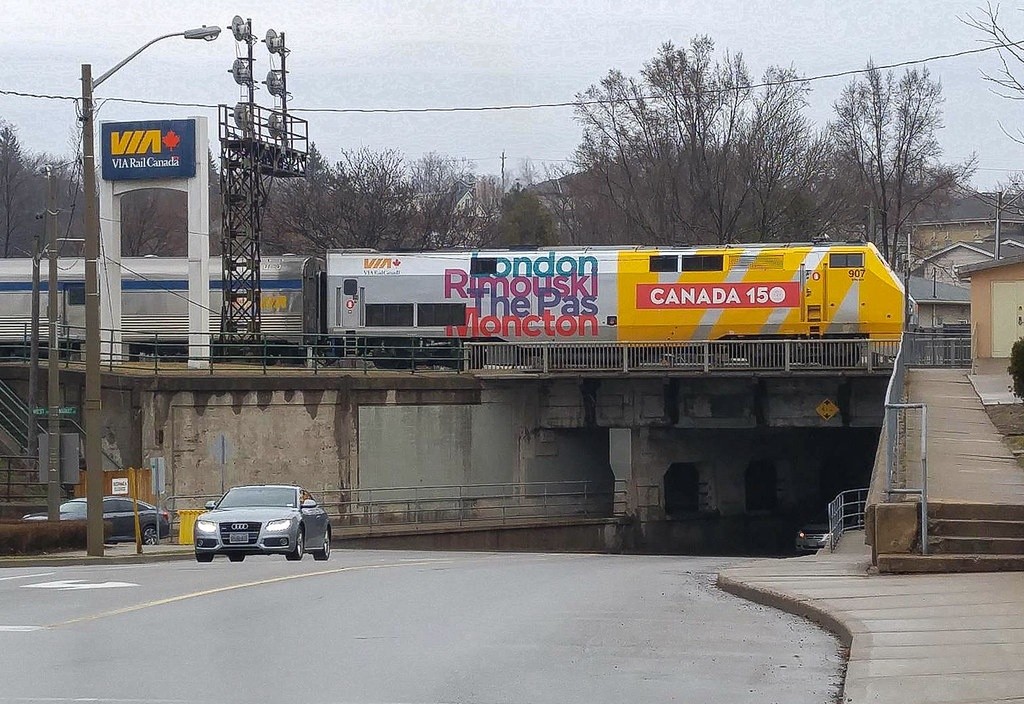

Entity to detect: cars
[795,514,831,555]
[20,496,170,545]
[193,483,332,563]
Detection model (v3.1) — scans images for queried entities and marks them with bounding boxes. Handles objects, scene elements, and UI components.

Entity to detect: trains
[1,248,922,368]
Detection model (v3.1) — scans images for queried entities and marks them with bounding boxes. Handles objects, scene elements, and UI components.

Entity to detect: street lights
[81,25,222,556]
